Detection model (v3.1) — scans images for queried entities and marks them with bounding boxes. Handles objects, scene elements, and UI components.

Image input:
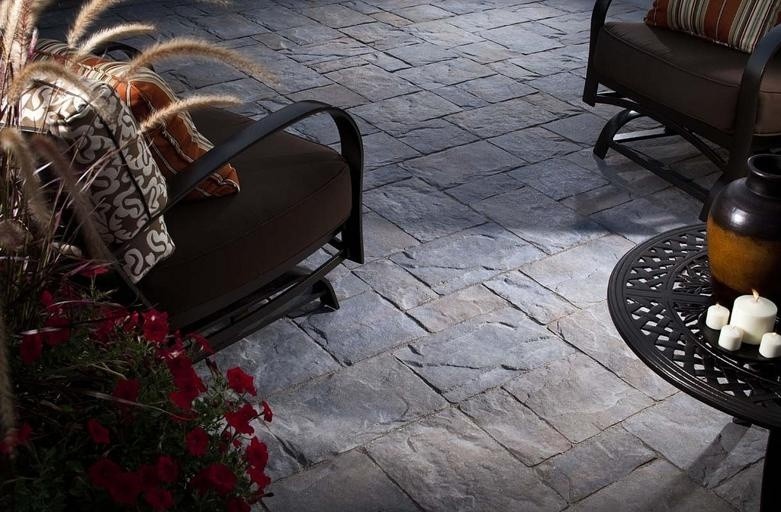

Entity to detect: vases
[706,155,780,301]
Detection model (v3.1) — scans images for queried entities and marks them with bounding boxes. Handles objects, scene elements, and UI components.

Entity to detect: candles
[705,288,781,359]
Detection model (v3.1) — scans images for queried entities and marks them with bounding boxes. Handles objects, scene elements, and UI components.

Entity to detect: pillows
[645,1,781,57]
[28,36,244,196]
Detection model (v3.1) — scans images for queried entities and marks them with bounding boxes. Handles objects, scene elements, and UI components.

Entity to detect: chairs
[1,40,365,367]
[581,0,781,221]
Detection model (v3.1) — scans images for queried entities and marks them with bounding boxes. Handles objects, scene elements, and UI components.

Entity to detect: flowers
[3,259,273,512]
